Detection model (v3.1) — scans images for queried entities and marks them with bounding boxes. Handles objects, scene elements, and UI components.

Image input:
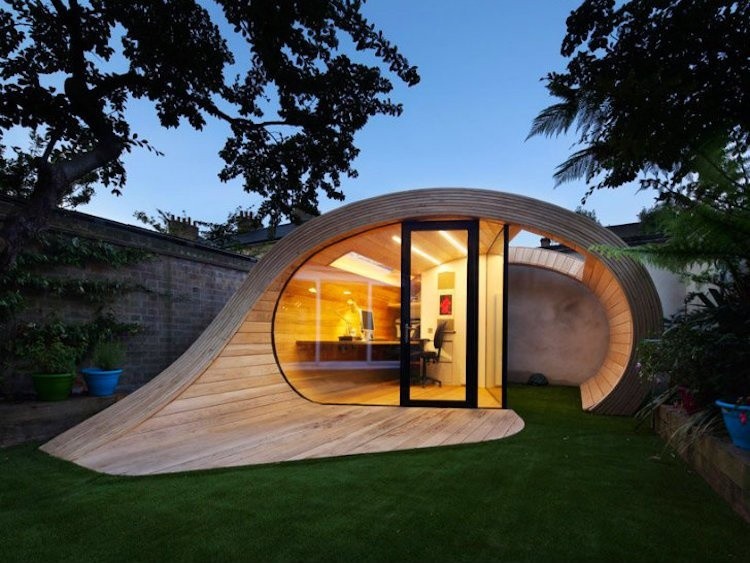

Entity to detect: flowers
[632,276,750,457]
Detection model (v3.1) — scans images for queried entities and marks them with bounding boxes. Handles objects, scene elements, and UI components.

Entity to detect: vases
[678,384,720,427]
[716,401,750,449]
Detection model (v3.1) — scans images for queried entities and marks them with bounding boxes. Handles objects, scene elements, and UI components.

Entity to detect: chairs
[410,322,446,389]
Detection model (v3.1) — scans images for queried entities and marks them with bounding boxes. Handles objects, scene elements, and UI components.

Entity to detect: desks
[296,340,421,346]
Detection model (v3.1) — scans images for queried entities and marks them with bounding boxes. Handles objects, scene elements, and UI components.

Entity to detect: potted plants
[18,333,78,401]
[80,336,129,396]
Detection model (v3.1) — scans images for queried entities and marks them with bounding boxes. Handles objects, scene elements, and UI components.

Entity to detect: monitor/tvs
[360,310,374,333]
